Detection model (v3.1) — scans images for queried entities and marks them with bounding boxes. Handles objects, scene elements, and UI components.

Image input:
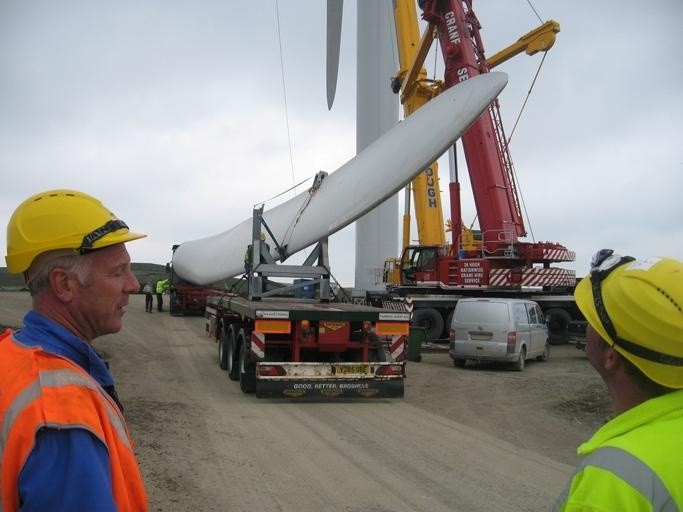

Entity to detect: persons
[560,249,683,512]
[156,278,169,312]
[144,281,153,313]
[0,188,149,512]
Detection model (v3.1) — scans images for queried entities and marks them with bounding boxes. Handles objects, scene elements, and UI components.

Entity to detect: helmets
[575,248,683,389]
[5,189,147,274]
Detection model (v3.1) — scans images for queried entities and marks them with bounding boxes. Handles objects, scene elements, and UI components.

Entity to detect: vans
[449,296,551,373]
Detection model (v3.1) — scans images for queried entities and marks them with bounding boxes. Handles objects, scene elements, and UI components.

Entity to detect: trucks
[166,244,412,400]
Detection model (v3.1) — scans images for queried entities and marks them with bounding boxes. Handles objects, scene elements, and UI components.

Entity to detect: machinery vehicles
[365,0,586,346]
